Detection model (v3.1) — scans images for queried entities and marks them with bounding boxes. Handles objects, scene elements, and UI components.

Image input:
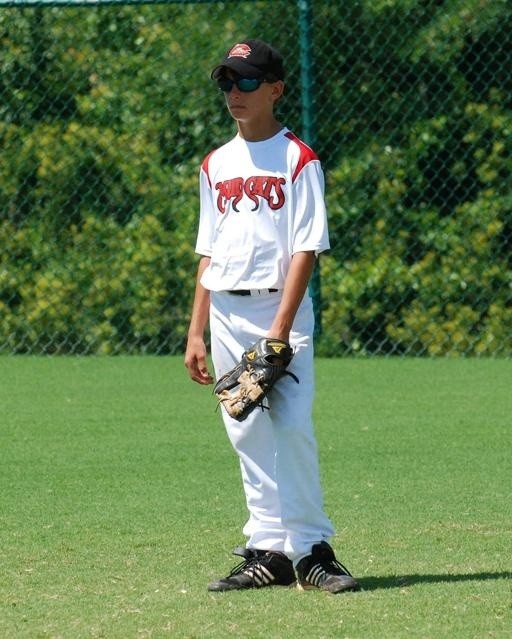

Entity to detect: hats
[211,39,285,83]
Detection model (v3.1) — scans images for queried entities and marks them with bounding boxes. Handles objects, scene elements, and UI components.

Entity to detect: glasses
[217,74,267,93]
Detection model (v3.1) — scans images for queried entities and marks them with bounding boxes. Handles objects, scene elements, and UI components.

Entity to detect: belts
[227,288,278,296]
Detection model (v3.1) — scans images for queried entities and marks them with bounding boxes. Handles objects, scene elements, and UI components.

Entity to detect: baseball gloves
[213,338,293,421]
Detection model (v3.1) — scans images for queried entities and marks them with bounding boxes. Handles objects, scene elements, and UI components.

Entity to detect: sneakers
[207,541,358,593]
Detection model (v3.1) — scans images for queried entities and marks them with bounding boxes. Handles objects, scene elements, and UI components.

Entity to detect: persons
[184,40,360,596]
